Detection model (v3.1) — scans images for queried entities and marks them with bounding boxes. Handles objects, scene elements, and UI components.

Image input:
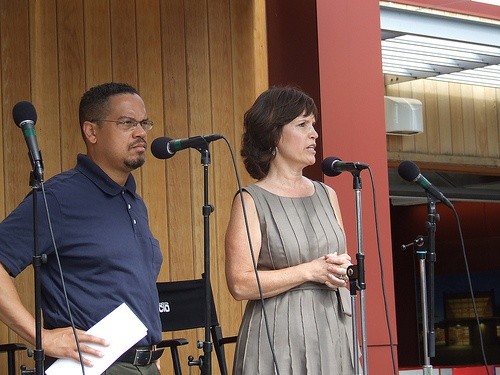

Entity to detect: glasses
[90,116,154,132]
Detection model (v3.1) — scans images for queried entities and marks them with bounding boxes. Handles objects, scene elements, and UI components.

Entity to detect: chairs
[0,273,237,375]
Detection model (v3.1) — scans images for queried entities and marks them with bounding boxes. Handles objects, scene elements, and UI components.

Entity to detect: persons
[0,83,164,375]
[224,87,364,375]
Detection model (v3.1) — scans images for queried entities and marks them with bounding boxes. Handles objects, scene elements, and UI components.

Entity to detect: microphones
[12,101,44,182]
[322,157,369,177]
[398,160,453,208]
[151,133,224,159]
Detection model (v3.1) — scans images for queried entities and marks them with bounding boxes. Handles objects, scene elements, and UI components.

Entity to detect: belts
[121,346,166,367]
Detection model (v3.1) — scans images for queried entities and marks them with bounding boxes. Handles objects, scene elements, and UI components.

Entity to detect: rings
[338,274,344,280]
